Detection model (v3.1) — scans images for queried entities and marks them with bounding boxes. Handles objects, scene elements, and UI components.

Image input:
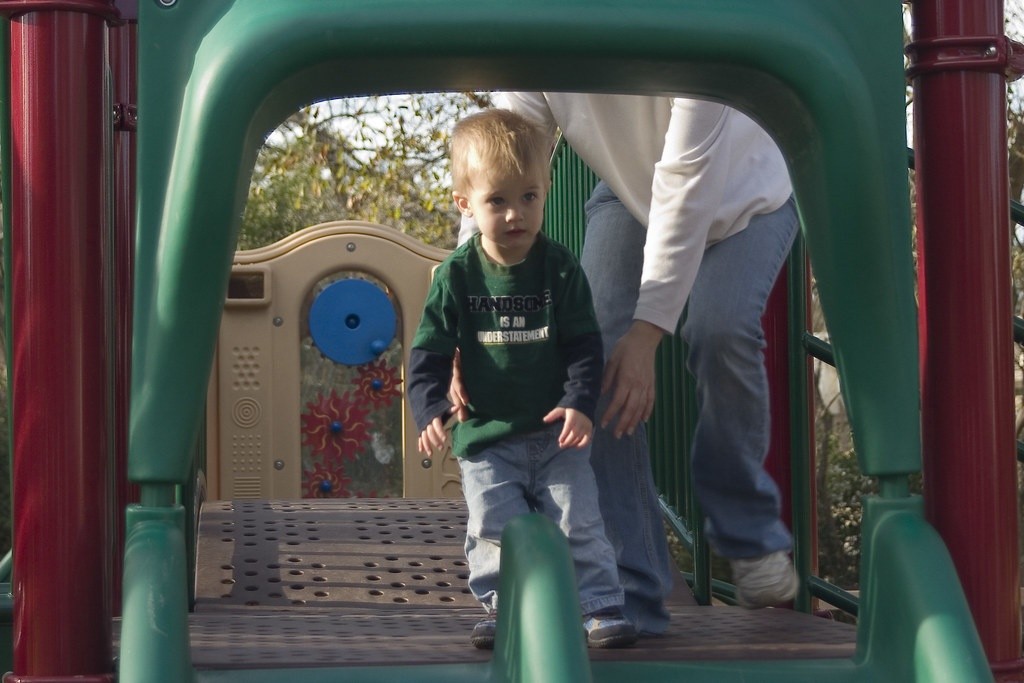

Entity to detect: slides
[118,501,997,683]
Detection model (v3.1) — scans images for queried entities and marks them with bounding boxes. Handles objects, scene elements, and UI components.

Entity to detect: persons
[405,110,636,649]
[431,87,804,642]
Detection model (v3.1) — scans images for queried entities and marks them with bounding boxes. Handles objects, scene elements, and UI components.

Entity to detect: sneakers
[469,608,499,650]
[582,604,637,648]
[731,550,801,610]
